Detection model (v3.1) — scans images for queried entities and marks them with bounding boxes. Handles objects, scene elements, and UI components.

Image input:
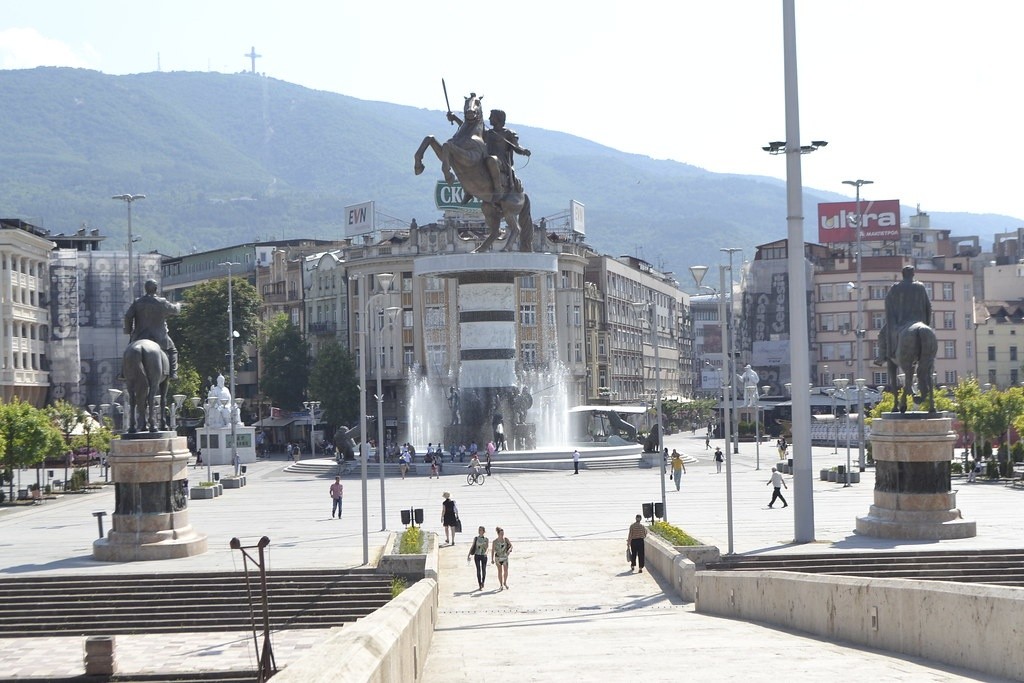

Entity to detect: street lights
[190,396,218,485]
[980,382,993,455]
[690,248,744,555]
[112,191,147,307]
[832,378,855,486]
[748,384,770,470]
[220,258,240,475]
[705,361,721,439]
[153,393,186,431]
[760,138,827,544]
[88,388,137,475]
[855,376,868,472]
[302,400,321,456]
[839,177,872,473]
[376,307,403,530]
[220,399,245,476]
[632,301,672,522]
[897,372,937,412]
[359,272,396,562]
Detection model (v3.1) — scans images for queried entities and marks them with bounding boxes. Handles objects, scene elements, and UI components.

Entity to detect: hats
[716,447,720,450]
[443,492,450,497]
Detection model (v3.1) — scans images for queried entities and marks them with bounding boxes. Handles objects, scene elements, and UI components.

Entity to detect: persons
[572,449,580,474]
[208,372,245,428]
[692,421,724,474]
[663,448,686,491]
[766,467,788,509]
[968,457,977,482]
[194,448,203,469]
[467,526,513,591]
[321,439,344,465]
[441,491,458,546]
[329,476,343,520]
[873,265,933,366]
[449,441,494,482]
[446,109,531,205]
[446,386,533,426]
[736,364,759,407]
[287,441,301,463]
[366,438,442,480]
[626,515,647,573]
[116,279,181,380]
[776,438,789,460]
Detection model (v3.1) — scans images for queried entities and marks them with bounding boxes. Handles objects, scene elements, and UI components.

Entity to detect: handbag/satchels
[455,519,461,532]
[670,474,673,480]
[471,537,478,555]
[627,547,631,562]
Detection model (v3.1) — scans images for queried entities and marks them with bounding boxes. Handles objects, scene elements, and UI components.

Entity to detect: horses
[414,92,534,253]
[122,338,170,432]
[886,322,938,413]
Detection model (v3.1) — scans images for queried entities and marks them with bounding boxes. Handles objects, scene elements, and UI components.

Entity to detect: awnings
[251,416,294,427]
[713,389,883,410]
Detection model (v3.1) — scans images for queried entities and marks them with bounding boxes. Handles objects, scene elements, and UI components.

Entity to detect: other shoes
[504,583,508,589]
[632,565,634,570]
[782,504,788,508]
[445,540,449,543]
[639,570,642,573]
[768,504,772,508]
[500,585,503,590]
[452,542,454,545]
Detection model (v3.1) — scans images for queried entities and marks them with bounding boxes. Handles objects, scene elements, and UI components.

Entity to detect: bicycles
[466,466,485,486]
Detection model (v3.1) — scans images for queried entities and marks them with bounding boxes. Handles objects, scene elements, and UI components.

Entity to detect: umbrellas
[568,404,652,436]
